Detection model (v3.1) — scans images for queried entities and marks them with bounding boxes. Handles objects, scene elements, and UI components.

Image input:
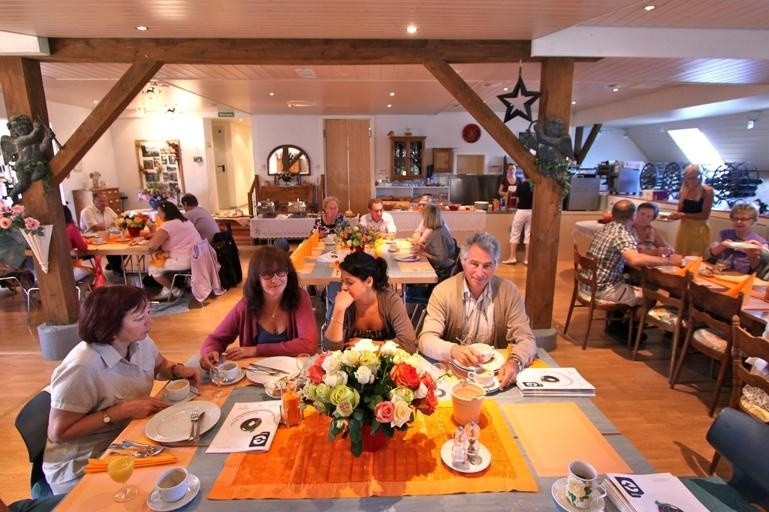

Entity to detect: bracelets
[686,212,689,218]
[171,362,184,380]
[509,357,522,372]
[331,317,343,325]
[667,255,671,265]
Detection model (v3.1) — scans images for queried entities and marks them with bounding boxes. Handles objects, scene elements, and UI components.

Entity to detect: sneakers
[502,259,516,264]
[172,288,183,298]
[154,286,172,300]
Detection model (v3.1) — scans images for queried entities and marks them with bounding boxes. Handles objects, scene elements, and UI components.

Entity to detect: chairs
[705,313,769,478]
[677,405,769,511]
[1,201,491,337]
[671,275,744,415]
[15,383,55,501]
[562,244,633,351]
[749,243,768,281]
[632,262,692,383]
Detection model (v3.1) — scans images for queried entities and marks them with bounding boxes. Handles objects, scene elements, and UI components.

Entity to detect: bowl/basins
[382,187,522,213]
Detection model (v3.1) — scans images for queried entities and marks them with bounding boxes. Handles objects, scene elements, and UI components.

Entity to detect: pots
[254,199,275,215]
[287,201,307,213]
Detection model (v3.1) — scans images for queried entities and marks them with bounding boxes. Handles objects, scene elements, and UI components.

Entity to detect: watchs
[100,409,112,425]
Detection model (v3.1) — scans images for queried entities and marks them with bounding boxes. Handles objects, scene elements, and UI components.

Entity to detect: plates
[452,345,505,395]
[166,388,197,400]
[145,474,203,510]
[656,210,677,222]
[211,208,250,219]
[438,437,493,474]
[213,369,246,386]
[143,401,222,446]
[80,224,152,248]
[551,477,608,512]
[246,356,301,384]
[318,228,420,265]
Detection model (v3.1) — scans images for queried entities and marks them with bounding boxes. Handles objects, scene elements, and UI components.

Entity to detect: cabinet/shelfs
[70,186,122,227]
[563,157,646,213]
[261,185,315,212]
[389,136,428,180]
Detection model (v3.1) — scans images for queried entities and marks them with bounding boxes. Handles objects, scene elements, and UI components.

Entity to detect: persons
[79,191,125,277]
[41,285,202,496]
[497,163,521,208]
[200,246,319,370]
[501,173,532,265]
[181,193,220,239]
[310,197,348,235]
[703,203,768,274]
[0,112,55,198]
[407,195,432,242]
[61,204,96,308]
[320,250,418,356]
[418,232,537,388]
[360,197,397,239]
[408,206,456,304]
[146,201,202,301]
[747,237,769,282]
[658,164,714,256]
[580,199,657,344]
[622,201,675,329]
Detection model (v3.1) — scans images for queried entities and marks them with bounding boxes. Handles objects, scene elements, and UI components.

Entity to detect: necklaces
[266,304,280,319]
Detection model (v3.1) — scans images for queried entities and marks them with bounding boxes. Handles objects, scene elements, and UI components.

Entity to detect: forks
[187,410,198,440]
[240,367,280,376]
[107,437,165,460]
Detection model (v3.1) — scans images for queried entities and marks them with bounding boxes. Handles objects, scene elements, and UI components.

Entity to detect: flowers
[133,183,183,200]
[300,338,439,453]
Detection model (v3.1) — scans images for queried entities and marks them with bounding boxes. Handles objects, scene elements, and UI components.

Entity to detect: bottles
[468,419,482,461]
[376,142,445,188]
[452,423,467,467]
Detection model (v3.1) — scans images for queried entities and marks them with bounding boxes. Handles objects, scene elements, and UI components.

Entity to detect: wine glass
[107,451,142,502]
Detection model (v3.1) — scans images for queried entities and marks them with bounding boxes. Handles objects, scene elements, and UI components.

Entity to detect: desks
[571,218,606,248]
[375,183,451,203]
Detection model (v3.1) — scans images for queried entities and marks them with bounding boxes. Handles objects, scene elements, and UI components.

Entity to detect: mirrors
[267,145,313,177]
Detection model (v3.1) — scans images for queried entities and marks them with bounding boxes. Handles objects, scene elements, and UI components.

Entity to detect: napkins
[675,254,704,278]
[726,270,757,307]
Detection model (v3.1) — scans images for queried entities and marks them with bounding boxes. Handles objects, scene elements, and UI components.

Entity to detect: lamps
[134,79,180,117]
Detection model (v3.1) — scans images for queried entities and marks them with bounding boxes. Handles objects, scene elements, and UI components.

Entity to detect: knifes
[193,411,206,443]
[249,363,291,375]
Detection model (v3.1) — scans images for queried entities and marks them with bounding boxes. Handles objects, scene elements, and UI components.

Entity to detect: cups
[280,381,304,428]
[296,353,312,378]
[639,243,751,291]
[214,361,240,380]
[450,381,486,425]
[154,465,191,502]
[167,379,191,397]
[565,460,607,508]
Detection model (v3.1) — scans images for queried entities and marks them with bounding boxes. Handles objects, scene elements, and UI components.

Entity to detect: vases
[147,195,166,209]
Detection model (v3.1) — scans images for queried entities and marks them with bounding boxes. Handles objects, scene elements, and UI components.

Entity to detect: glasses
[261,269,286,280]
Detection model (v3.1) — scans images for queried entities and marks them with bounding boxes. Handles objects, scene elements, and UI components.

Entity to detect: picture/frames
[432,147,454,173]
[135,137,186,201]
